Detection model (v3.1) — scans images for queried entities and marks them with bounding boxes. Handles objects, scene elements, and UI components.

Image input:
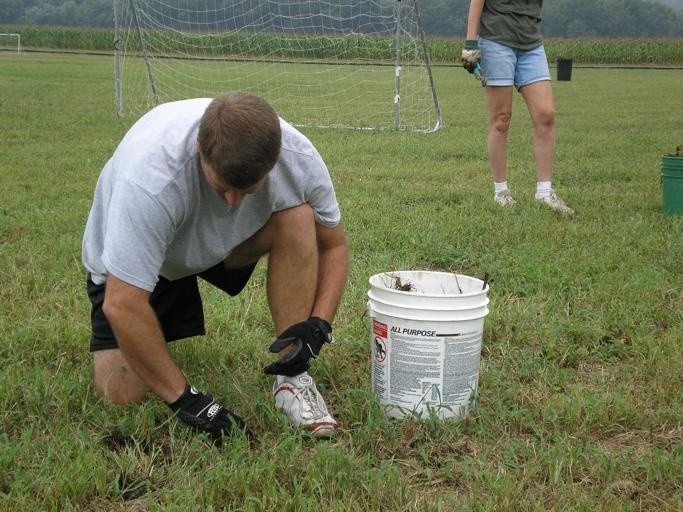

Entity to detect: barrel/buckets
[360,271,490,421]
[660,154,683,213]
[556,58,572,81]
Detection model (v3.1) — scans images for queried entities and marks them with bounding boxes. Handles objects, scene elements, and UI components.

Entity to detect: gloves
[167,383,249,440]
[461,40,481,73]
[264,317,332,376]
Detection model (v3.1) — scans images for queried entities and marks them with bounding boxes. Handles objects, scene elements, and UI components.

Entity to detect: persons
[460,1,579,216]
[83,91,348,441]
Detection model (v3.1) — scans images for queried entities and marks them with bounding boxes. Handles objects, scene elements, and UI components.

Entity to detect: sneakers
[272,373,338,439]
[534,189,574,215]
[494,189,516,209]
